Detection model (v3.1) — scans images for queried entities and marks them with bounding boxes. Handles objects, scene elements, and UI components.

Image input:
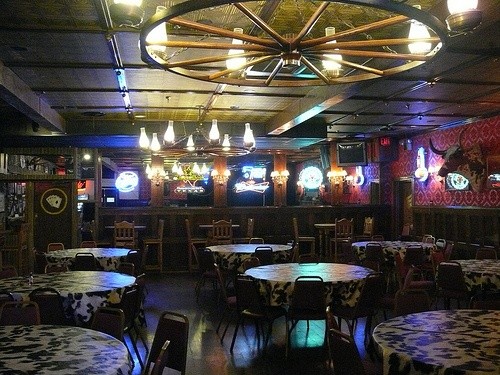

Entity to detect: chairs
[0,215,500,375]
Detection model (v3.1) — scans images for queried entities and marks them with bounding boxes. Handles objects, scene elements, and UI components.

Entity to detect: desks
[49,247,137,259]
[206,243,293,255]
[373,309,500,375]
[104,225,147,230]
[0,270,136,300]
[351,241,434,255]
[314,223,336,257]
[245,262,376,287]
[199,224,240,228]
[440,259,500,281]
[0,325,130,375]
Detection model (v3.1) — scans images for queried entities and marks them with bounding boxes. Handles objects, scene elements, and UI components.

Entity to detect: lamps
[77,0,482,194]
